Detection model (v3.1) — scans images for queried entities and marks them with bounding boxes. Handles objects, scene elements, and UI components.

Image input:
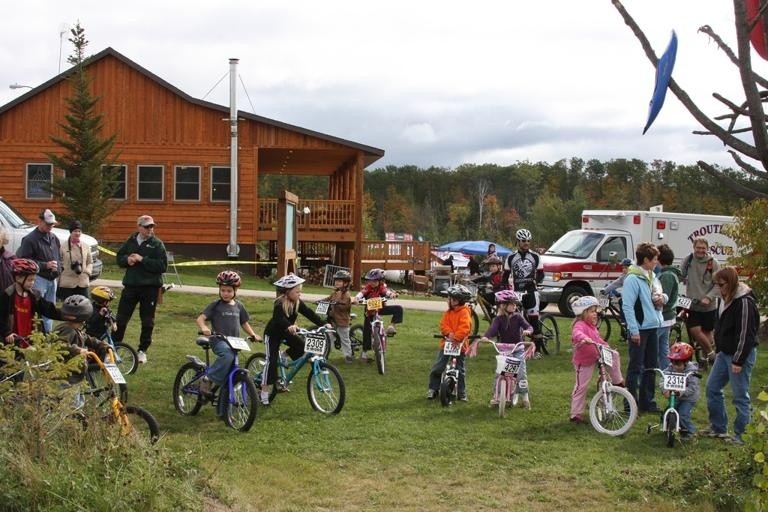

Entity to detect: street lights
[58,29,67,72]
[9,82,33,89]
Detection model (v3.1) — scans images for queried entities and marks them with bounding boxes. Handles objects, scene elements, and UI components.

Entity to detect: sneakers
[137,350,147,363]
[344,356,352,364]
[679,428,745,445]
[428,389,439,400]
[640,401,663,415]
[532,352,544,359]
[457,396,467,402]
[359,351,368,364]
[569,415,586,423]
[387,325,396,334]
[698,357,708,373]
[521,400,531,410]
[707,351,717,368]
[279,350,287,364]
[260,390,269,405]
[198,376,212,396]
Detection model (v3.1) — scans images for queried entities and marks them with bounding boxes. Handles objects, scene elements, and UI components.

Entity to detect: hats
[137,215,156,227]
[38,208,57,224]
[70,220,81,233]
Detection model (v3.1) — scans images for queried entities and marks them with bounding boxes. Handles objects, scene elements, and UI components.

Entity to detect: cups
[650,296,663,308]
[51,260,57,273]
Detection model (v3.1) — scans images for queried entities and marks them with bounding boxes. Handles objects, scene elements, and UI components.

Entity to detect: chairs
[161,251,182,286]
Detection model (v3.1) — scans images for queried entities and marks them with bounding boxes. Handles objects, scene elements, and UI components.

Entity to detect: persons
[464,256,512,324]
[315,270,354,365]
[56,220,94,304]
[620,242,670,417]
[656,244,682,374]
[678,237,723,362]
[658,341,701,444]
[262,274,334,407]
[194,271,263,398]
[479,290,535,412]
[353,268,404,365]
[600,259,633,327]
[47,294,109,412]
[0,258,68,384]
[14,208,65,335]
[500,228,545,361]
[426,283,473,403]
[439,244,498,276]
[84,287,119,383]
[568,296,626,423]
[0,224,17,346]
[113,215,168,364]
[699,267,760,446]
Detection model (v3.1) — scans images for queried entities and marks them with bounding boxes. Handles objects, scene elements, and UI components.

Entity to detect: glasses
[717,282,727,287]
[521,240,529,242]
[143,224,153,229]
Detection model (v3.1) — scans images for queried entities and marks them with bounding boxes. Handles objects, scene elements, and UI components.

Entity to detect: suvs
[0,192,104,286]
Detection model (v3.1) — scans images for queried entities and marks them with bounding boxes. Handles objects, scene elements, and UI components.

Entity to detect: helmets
[11,258,117,322]
[215,271,240,287]
[332,270,351,281]
[666,342,693,360]
[494,289,518,303]
[364,268,385,280]
[277,275,305,293]
[439,284,471,304]
[484,256,502,265]
[515,229,531,240]
[569,295,599,315]
[618,258,632,266]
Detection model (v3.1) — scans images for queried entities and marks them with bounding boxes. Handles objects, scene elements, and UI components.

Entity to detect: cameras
[71,260,83,275]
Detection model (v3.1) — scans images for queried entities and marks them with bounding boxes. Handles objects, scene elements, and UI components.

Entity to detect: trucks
[528,208,768,322]
